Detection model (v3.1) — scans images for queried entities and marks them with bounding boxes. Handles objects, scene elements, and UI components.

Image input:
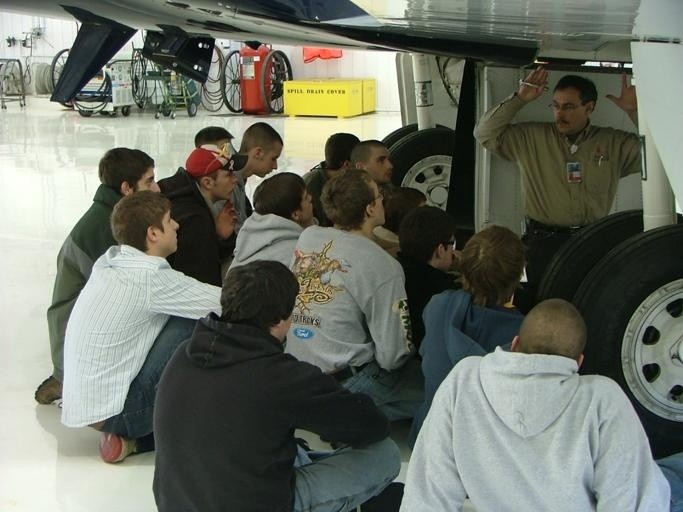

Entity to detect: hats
[185,143,249,175]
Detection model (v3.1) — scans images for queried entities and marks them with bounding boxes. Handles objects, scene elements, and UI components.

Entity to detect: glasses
[549,102,583,110]
[375,192,384,200]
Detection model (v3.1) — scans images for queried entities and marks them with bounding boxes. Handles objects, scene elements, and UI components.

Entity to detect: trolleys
[142,29,198,120]
[73,56,138,116]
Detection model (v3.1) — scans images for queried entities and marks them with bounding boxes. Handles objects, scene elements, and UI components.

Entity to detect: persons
[157,144,249,287]
[35,147,160,405]
[231,123,284,225]
[395,206,463,349]
[372,187,428,256]
[302,133,361,228]
[283,169,425,429]
[351,140,394,181]
[152,260,405,511]
[398,298,682,511]
[473,66,640,312]
[226,172,319,277]
[60,189,221,464]
[407,225,526,448]
[195,127,234,152]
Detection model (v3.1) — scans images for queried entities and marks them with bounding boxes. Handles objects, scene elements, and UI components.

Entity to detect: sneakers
[354,482,405,511]
[99,433,140,464]
[35,376,62,403]
